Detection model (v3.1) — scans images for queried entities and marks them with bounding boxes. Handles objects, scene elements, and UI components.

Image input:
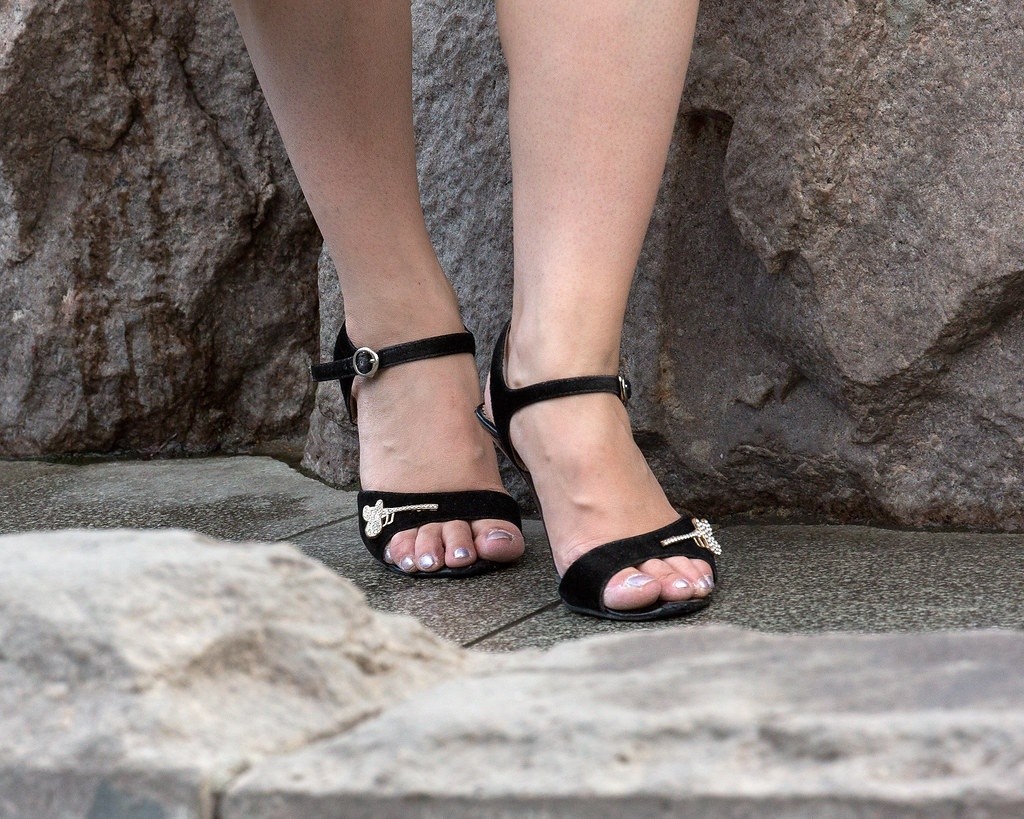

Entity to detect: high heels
[310,318,525,579]
[475,318,722,620]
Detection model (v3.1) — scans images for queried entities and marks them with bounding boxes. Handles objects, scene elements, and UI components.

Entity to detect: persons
[231,0,722,620]
